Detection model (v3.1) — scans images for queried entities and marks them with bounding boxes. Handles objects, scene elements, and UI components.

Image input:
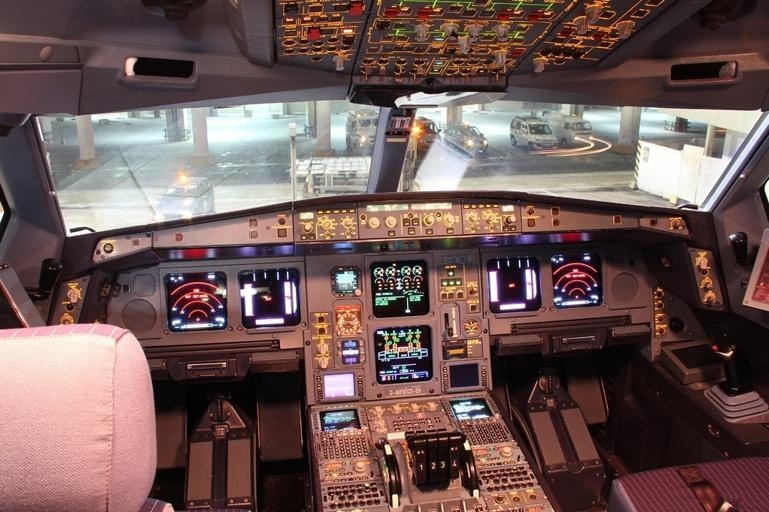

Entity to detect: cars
[444,124,488,153]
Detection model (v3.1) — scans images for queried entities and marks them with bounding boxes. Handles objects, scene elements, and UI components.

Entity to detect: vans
[345,110,380,152]
[509,115,558,150]
[154,176,214,221]
[417,117,441,153]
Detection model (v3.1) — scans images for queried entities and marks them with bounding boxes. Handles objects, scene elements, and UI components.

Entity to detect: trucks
[538,109,593,147]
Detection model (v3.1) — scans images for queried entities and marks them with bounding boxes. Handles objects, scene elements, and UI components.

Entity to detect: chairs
[2,324,180,510]
